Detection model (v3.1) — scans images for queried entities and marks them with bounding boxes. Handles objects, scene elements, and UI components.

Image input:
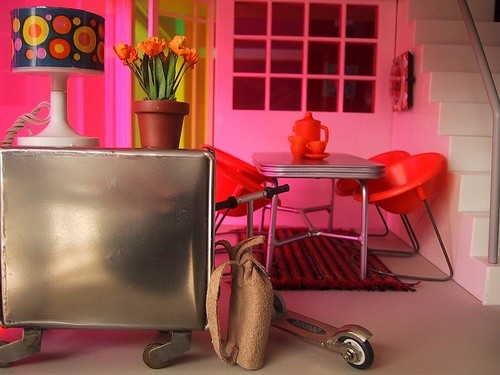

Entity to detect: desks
[253,149,385,280]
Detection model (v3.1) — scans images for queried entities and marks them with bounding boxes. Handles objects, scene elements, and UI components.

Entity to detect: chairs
[353,153,455,282]
[335,149,418,258]
[214,160,283,277]
[201,145,276,184]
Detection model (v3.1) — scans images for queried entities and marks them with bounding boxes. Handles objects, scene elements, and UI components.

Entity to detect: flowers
[109,34,200,100]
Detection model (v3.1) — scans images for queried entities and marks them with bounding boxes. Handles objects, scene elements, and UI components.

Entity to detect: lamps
[16,4,105,145]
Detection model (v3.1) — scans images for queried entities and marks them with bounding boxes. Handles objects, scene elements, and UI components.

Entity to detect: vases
[133,100,190,149]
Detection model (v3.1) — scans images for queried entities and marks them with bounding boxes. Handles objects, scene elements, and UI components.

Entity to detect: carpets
[237,227,416,291]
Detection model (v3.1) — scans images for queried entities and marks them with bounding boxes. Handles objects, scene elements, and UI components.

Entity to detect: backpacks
[206,234,277,370]
